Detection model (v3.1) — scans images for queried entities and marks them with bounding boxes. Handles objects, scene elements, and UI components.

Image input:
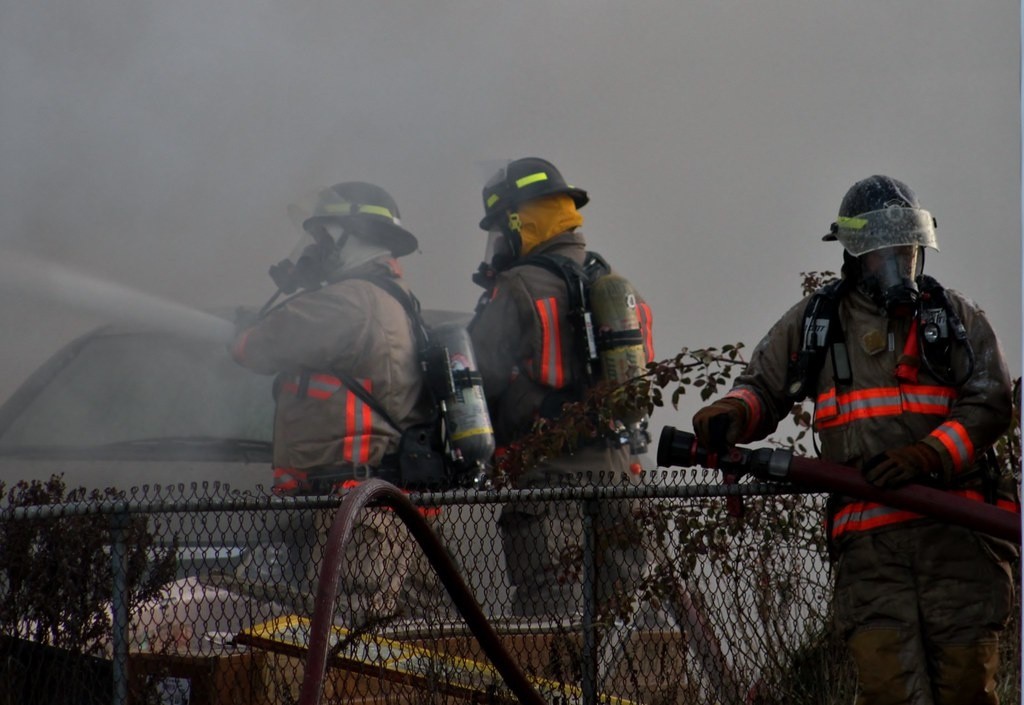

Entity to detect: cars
[1,308,475,600]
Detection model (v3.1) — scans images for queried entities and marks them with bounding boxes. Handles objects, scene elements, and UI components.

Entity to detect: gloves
[693,398,752,446]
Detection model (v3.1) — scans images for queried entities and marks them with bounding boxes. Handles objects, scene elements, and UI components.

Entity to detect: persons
[463,156,658,629]
[228,181,433,625]
[692,173,1021,704]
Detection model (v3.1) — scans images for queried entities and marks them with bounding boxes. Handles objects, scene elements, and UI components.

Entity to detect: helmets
[861,441,944,491]
[821,175,942,257]
[479,157,590,231]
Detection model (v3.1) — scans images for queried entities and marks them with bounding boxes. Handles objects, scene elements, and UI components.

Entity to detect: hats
[303,182,418,258]
